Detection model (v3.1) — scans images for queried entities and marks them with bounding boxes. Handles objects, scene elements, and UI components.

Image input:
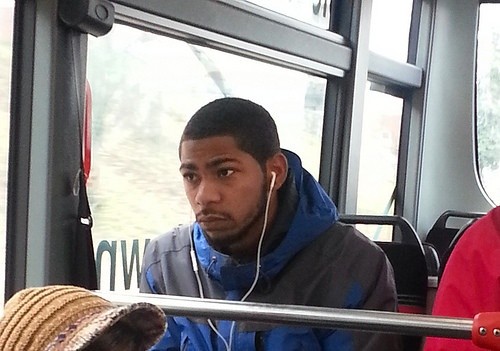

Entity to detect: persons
[137,97,402,351]
[424,206,500,351]
[0,285,167,351]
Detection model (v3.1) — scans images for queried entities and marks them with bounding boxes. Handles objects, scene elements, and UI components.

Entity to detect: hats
[0,285,168,351]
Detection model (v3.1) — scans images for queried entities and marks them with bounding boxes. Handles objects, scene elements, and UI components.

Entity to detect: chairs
[426,210,487,260]
[337,214,428,351]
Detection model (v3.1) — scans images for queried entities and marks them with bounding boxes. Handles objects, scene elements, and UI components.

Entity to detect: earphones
[269,171,276,190]
